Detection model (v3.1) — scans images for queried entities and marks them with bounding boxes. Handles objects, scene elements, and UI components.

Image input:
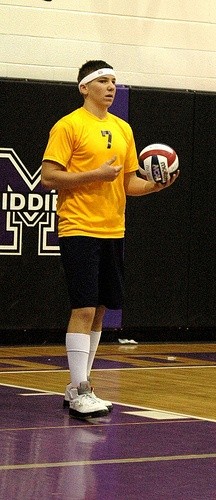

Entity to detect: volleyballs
[138,143,178,185]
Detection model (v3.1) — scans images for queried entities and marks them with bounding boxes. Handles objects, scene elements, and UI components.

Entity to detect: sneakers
[64,381,113,419]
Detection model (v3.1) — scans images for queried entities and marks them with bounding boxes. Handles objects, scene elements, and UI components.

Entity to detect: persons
[40,58,181,419]
